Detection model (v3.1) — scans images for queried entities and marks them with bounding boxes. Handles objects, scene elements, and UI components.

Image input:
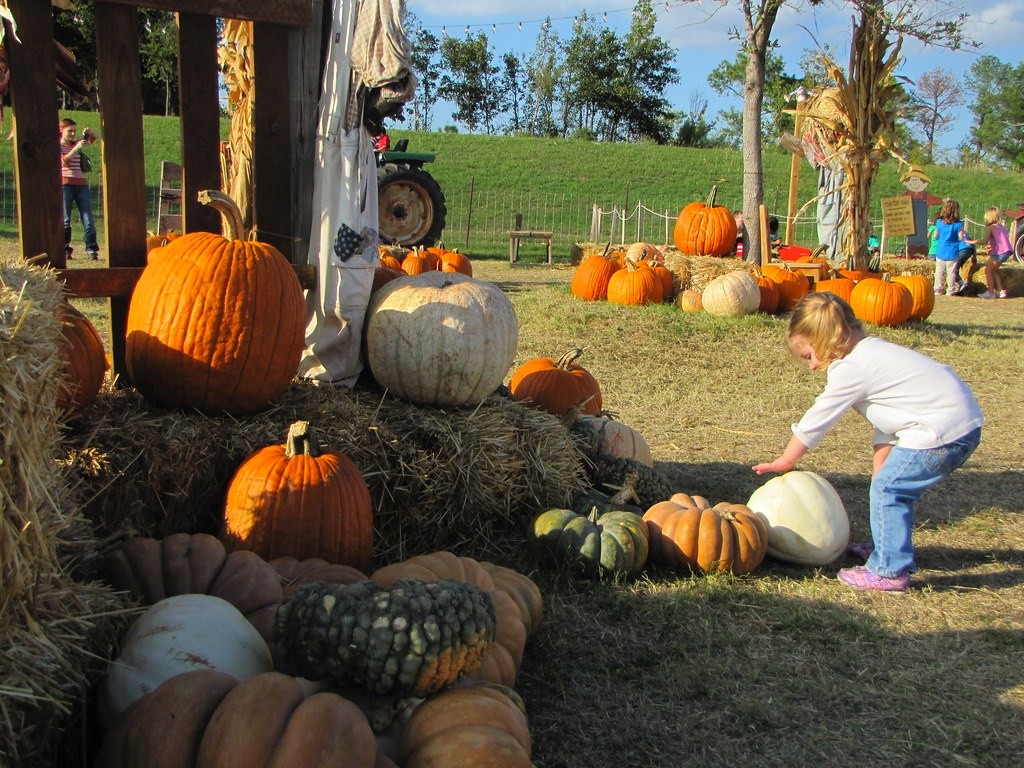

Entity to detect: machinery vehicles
[376,139,447,250]
[1003,203,1024,262]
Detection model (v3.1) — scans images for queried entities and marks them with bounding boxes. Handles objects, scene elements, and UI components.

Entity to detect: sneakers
[844,541,874,560]
[837,564,911,592]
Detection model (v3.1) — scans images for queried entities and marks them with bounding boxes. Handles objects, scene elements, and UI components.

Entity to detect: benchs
[509,230,553,267]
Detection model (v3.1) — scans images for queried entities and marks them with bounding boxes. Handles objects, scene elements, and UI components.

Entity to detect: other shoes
[947,291,957,296]
[957,283,969,295]
[994,289,1008,298]
[86,252,99,260]
[66,249,71,259]
[977,291,997,299]
[935,288,944,295]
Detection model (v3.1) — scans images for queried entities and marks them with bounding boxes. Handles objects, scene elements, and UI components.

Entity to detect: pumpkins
[49,188,306,418]
[90,419,546,768]
[571,185,934,327]
[362,244,850,577]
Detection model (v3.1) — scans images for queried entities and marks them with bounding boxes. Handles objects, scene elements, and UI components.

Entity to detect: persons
[752,291,983,594]
[925,200,977,295]
[868,222,879,257]
[769,216,782,248]
[964,207,1013,298]
[377,128,390,153]
[734,211,744,257]
[60,118,100,260]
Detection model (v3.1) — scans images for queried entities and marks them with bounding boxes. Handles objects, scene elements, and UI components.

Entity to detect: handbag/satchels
[78,149,92,173]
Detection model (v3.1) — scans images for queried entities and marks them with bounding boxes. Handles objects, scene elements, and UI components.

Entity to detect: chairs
[759,203,824,283]
